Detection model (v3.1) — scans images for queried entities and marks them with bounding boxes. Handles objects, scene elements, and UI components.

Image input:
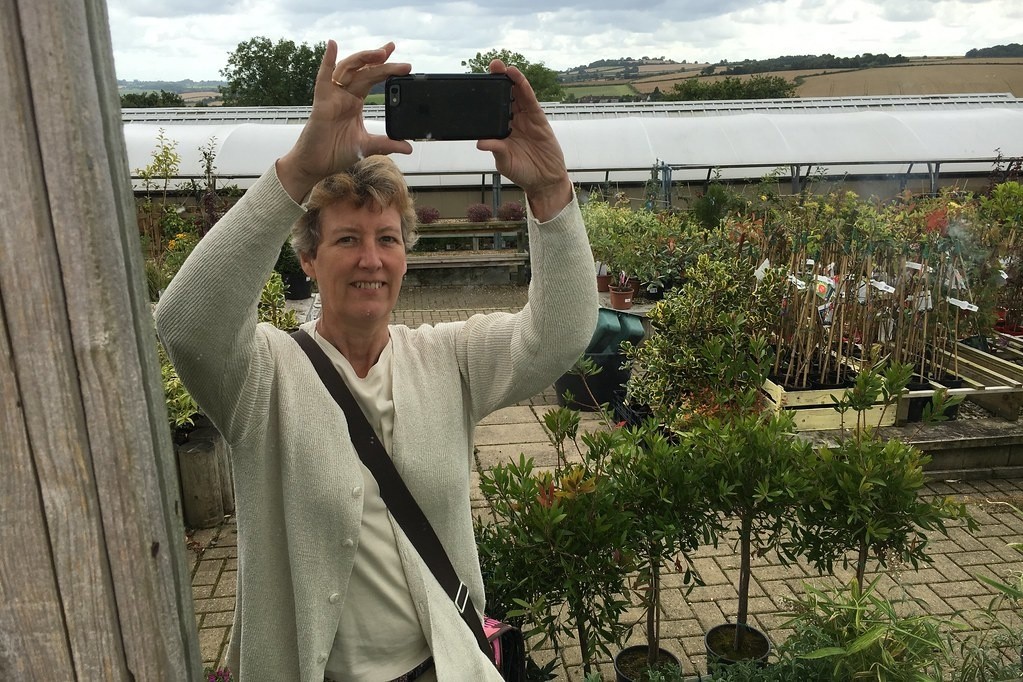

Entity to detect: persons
[154,41,599,682]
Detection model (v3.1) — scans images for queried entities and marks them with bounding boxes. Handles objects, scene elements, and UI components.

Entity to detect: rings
[332,78,347,89]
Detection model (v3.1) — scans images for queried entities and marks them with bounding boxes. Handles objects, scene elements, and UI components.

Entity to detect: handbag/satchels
[482,615,525,682]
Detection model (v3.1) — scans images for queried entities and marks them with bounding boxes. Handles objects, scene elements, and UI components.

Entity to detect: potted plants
[125,135,1023,682]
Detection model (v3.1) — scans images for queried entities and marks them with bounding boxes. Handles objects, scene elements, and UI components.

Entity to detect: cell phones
[385,73,515,142]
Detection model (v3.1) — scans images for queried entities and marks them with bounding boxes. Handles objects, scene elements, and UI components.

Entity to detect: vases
[555,370,631,413]
[584,309,643,354]
